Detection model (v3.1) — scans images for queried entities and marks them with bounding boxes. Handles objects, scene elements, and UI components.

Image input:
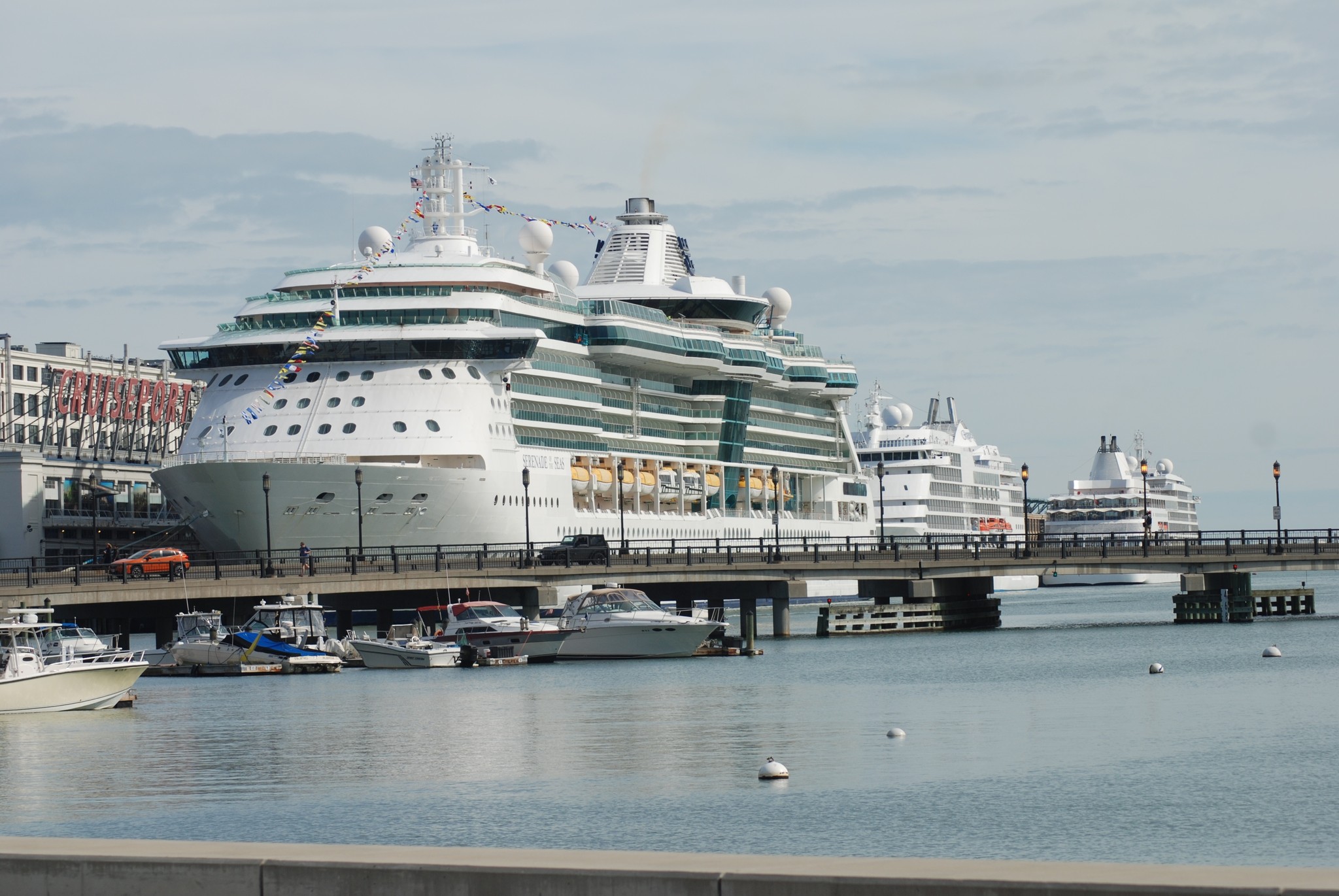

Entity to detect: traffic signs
[772,514,779,525]
[1273,506,1280,519]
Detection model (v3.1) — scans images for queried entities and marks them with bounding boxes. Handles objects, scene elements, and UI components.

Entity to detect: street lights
[617,461,627,555]
[770,465,783,561]
[261,471,275,575]
[89,471,100,571]
[522,466,534,566]
[354,466,366,562]
[1141,458,1147,538]
[1021,461,1031,556]
[877,460,887,551]
[1273,459,1284,554]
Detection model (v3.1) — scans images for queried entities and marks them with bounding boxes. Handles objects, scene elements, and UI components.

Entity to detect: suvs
[109,547,190,578]
[537,534,609,566]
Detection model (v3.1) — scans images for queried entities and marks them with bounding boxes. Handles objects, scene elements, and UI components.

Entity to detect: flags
[410,177,422,189]
[1148,451,1152,456]
[487,176,497,185]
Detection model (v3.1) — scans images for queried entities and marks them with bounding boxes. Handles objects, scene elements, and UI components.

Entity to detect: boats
[570,466,590,492]
[151,132,876,627]
[1158,523,1168,535]
[999,519,1012,534]
[705,473,720,497]
[346,623,479,667]
[555,582,729,659]
[641,467,680,502]
[601,469,633,498]
[232,593,361,659]
[167,597,348,675]
[987,518,1003,534]
[131,555,230,665]
[414,549,586,664]
[736,476,763,501]
[0,617,123,665]
[624,472,655,499]
[979,518,990,535]
[667,469,703,504]
[752,478,780,502]
[580,468,612,496]
[0,609,149,715]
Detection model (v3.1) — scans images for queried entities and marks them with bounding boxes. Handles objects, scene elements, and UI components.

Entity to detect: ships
[848,378,1040,592]
[1042,434,1201,585]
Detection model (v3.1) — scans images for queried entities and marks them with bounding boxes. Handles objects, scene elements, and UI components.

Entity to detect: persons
[432,222,438,235]
[667,315,673,322]
[298,542,312,577]
[102,544,112,582]
[1141,511,1152,540]
[109,543,121,561]
[593,302,603,316]
